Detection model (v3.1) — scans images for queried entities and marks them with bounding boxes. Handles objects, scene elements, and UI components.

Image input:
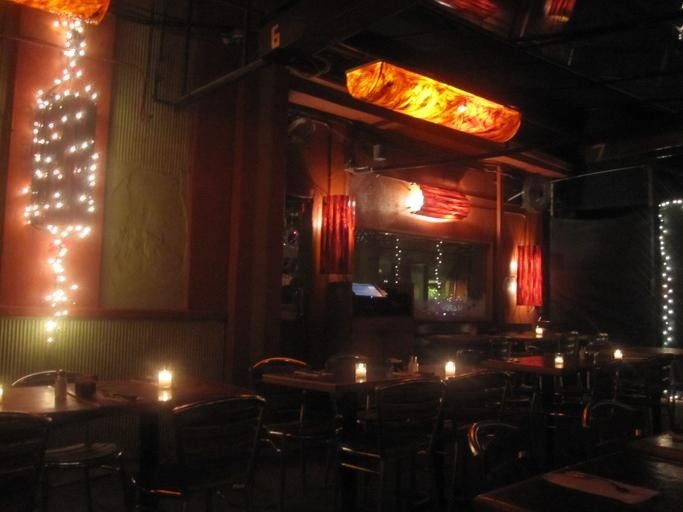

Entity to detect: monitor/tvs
[351,282,388,299]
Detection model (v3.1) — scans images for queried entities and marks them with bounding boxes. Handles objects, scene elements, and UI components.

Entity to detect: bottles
[408,354,419,374]
[55,369,67,402]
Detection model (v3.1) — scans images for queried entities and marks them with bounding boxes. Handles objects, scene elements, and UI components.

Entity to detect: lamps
[344,57,525,145]
[318,194,359,283]
[2,0,111,26]
[516,213,545,306]
[397,182,472,222]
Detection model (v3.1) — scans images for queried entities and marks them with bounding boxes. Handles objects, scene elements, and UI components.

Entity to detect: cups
[353,363,366,382]
[554,351,564,368]
[535,327,543,337]
